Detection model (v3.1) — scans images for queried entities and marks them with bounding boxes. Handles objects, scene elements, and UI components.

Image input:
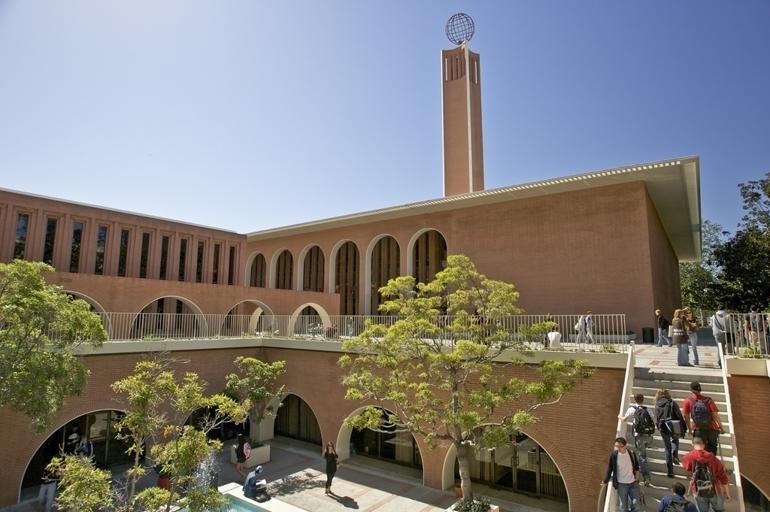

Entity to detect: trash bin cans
[643,327,654,344]
[454,483,462,498]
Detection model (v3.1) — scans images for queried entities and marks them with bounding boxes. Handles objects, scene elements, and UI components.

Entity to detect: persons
[599,437,644,511]
[652,389,688,477]
[322,442,339,495]
[242,466,271,500]
[78,437,98,469]
[681,437,730,510]
[153,459,172,490]
[617,394,658,487]
[232,433,249,484]
[472,304,768,371]
[682,381,724,457]
[37,464,55,512]
[64,424,78,454]
[657,481,696,512]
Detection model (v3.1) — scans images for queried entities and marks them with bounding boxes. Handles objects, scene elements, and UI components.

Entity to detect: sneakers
[673,455,679,465]
[644,477,651,487]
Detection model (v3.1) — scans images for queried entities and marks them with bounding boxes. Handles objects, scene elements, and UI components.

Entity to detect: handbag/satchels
[574,322,579,331]
[717,331,735,344]
[659,418,686,437]
[639,486,646,506]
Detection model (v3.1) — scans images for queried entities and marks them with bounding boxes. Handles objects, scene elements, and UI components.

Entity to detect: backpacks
[243,442,252,460]
[663,495,690,512]
[629,405,655,437]
[688,396,711,426]
[690,461,715,499]
